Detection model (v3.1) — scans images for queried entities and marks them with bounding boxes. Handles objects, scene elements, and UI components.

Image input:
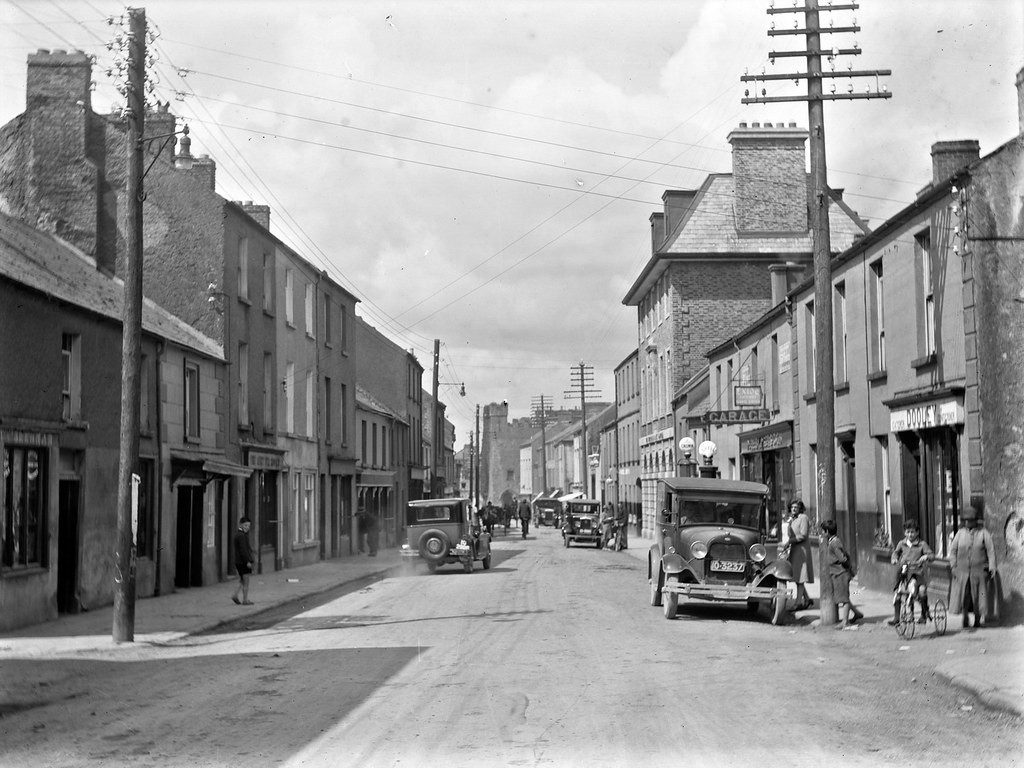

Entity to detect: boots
[888,600,901,625]
[918,595,928,622]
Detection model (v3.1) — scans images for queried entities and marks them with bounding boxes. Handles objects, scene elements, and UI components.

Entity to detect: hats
[961,506,979,520]
[522,499,527,503]
[356,507,366,513]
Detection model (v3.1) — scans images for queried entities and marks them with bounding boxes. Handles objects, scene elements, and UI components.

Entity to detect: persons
[948,507,998,632]
[231,517,254,606]
[679,500,864,629]
[479,493,532,539]
[599,501,628,551]
[358,507,381,557]
[885,517,934,624]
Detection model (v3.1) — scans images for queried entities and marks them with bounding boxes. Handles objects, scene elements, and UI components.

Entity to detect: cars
[646,477,793,626]
[561,498,603,547]
[397,498,492,571]
[532,498,561,528]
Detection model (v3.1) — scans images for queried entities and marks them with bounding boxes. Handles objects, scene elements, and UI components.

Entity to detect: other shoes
[849,613,864,623]
[243,600,254,605]
[973,616,981,628]
[602,547,610,550]
[834,622,847,631]
[232,596,241,604]
[963,617,969,628]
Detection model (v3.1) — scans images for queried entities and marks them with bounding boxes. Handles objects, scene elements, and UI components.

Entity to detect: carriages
[477,505,511,538]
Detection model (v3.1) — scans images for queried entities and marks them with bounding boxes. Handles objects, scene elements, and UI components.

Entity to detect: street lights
[674,436,698,478]
[474,430,497,513]
[109,125,199,643]
[430,382,466,498]
[605,373,621,523]
[590,461,598,500]
[698,440,720,480]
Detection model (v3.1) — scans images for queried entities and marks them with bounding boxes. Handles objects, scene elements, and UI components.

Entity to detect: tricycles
[892,560,948,641]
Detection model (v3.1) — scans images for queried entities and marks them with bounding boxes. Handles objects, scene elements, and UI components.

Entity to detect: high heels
[803,598,814,609]
[787,604,803,611]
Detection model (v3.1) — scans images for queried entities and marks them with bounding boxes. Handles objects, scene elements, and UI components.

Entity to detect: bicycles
[520,518,529,540]
[610,524,626,552]
